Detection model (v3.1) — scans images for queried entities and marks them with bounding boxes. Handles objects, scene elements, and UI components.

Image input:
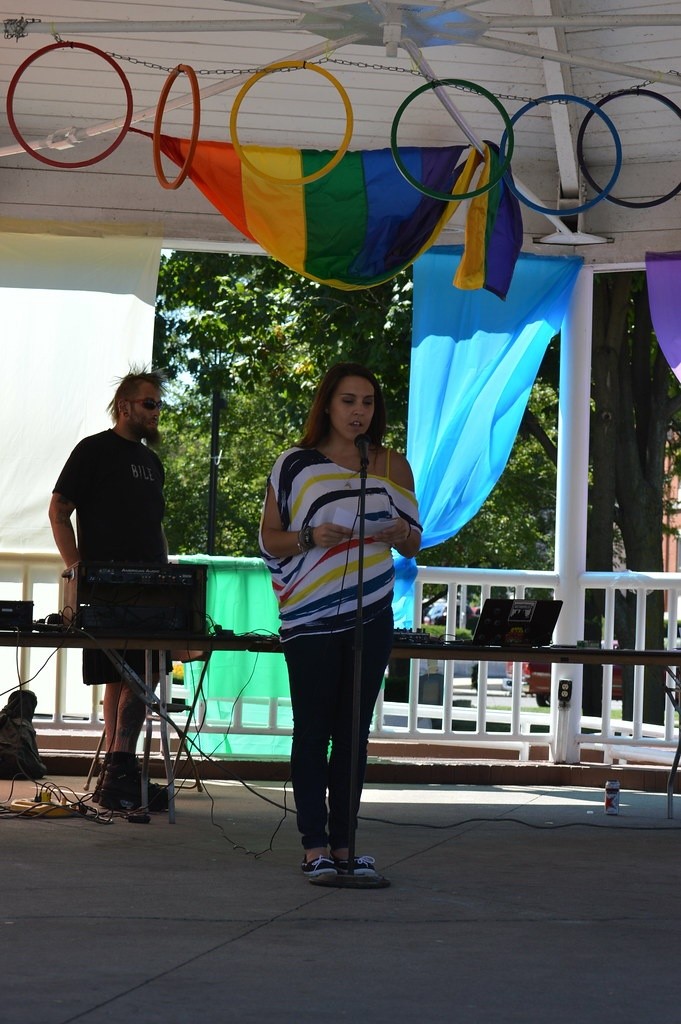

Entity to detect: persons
[256,363,422,884]
[48,374,168,812]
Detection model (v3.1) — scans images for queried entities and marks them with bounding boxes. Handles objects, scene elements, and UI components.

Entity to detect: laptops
[451,599,563,645]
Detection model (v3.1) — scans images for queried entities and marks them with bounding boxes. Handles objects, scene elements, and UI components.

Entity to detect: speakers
[64,562,207,638]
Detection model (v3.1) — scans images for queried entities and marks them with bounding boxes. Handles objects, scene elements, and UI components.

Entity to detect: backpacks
[0,690,47,779]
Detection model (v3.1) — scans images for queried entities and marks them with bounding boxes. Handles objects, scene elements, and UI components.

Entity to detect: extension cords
[12,797,77,817]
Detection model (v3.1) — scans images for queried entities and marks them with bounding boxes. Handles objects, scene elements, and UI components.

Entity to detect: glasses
[130,397,162,410]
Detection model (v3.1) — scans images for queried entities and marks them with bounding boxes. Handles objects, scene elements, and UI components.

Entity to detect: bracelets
[407,524,411,538]
[297,526,314,551]
[70,561,80,569]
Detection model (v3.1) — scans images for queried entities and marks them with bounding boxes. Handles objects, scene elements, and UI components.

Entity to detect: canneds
[603,779,620,815]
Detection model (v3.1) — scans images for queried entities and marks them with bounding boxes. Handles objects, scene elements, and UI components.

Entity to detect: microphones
[354,433,371,467]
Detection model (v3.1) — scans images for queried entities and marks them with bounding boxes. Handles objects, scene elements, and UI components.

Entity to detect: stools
[84,701,203,794]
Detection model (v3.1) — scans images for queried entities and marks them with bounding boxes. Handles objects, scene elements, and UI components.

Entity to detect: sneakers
[329,850,375,874]
[301,854,337,876]
[92,757,174,811]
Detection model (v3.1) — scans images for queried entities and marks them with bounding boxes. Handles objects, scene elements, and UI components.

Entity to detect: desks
[0,624,681,826]
